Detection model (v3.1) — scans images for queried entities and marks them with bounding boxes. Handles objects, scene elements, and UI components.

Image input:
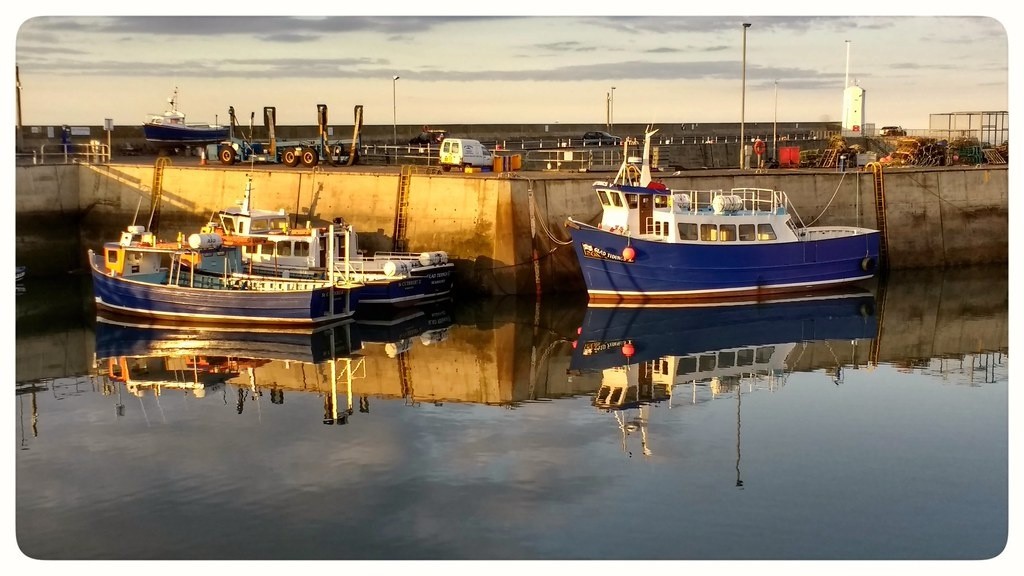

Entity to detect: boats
[144,87,230,145]
[89,178,455,325]
[564,124,884,300]
[564,285,883,460]
[89,300,455,428]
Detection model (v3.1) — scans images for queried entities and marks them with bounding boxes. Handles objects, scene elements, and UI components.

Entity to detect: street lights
[740,23,752,169]
[393,76,400,146]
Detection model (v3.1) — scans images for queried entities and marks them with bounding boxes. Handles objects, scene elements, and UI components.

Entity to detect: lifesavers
[754,141,766,154]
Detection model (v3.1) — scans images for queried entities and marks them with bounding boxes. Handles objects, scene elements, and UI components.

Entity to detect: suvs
[409,130,450,145]
[582,131,622,147]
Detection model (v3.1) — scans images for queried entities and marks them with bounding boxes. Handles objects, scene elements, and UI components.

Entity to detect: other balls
[622,248,635,259]
[622,344,635,356]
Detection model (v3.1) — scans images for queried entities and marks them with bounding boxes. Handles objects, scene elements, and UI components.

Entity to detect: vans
[439,137,498,173]
